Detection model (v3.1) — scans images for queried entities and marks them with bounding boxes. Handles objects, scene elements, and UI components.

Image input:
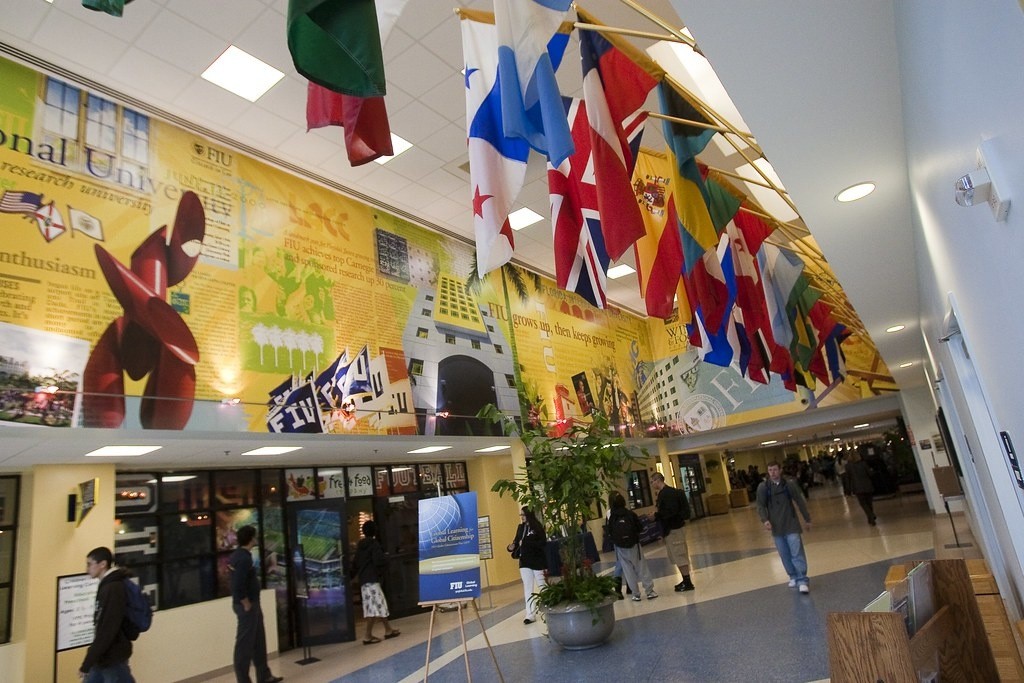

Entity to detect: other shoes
[869,515,876,525]
[675,581,695,591]
[798,585,809,592]
[647,590,658,598]
[632,595,641,600]
[260,675,283,683]
[523,619,533,625]
[788,578,797,588]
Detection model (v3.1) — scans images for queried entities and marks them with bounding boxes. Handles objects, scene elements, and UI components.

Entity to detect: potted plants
[475,377,647,653]
[706,460,720,471]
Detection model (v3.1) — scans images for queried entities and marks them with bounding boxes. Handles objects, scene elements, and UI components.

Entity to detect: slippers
[362,636,381,644]
[384,630,401,639]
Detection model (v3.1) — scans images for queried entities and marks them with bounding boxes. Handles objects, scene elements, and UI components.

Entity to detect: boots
[626,580,632,594]
[614,577,624,600]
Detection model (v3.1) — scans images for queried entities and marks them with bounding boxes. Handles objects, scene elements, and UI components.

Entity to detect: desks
[543,531,602,577]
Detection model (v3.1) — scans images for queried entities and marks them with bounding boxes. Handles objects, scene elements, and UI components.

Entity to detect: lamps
[954,143,1013,222]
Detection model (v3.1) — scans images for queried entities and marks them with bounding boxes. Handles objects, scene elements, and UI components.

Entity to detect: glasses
[519,513,527,517]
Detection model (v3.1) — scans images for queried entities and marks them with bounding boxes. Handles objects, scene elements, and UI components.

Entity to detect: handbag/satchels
[511,543,521,559]
[602,524,614,553]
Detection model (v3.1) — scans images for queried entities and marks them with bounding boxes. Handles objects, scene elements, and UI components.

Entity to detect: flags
[82,0,849,393]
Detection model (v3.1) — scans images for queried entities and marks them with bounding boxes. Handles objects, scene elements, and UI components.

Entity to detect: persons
[649,473,694,592]
[507,506,547,623]
[229,526,283,683]
[845,453,877,527]
[78,547,139,683]
[756,461,810,593]
[605,492,658,600]
[401,525,416,546]
[728,448,856,498]
[353,520,400,644]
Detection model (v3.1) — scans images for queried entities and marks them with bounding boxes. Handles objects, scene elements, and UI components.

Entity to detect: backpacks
[611,509,641,548]
[121,577,156,642]
[656,487,691,521]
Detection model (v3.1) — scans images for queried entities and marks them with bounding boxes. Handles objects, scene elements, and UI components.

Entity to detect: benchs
[633,513,661,544]
